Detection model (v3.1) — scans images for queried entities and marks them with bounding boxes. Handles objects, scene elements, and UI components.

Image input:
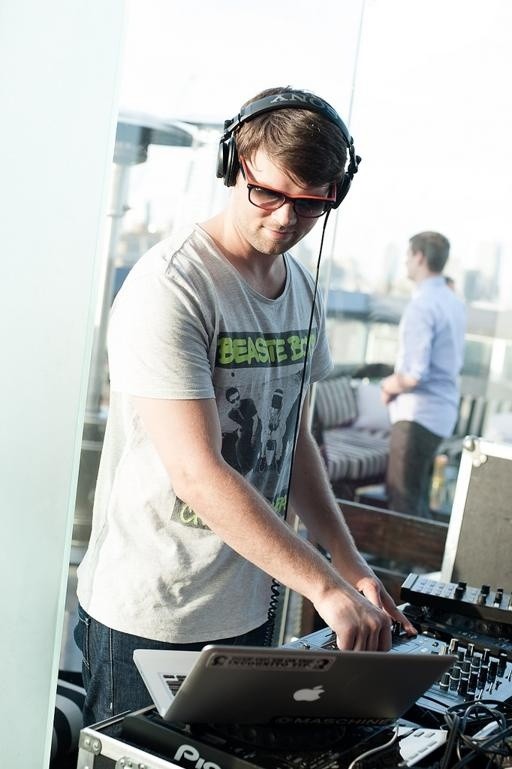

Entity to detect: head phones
[216,90,361,209]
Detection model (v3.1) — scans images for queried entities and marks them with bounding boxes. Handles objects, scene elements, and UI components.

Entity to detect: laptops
[132,644,458,723]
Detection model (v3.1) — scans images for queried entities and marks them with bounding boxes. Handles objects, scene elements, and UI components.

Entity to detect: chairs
[47,667,95,762]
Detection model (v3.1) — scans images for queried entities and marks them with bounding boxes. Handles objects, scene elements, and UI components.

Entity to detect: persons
[70,85,420,734]
[379,231,467,521]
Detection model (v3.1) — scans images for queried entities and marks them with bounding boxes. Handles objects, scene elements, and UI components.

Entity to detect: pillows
[351,379,394,436]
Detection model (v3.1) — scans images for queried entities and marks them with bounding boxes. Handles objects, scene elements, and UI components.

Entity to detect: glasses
[238,154,338,220]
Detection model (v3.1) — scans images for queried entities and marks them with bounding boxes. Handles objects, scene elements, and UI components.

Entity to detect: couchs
[309,371,509,487]
